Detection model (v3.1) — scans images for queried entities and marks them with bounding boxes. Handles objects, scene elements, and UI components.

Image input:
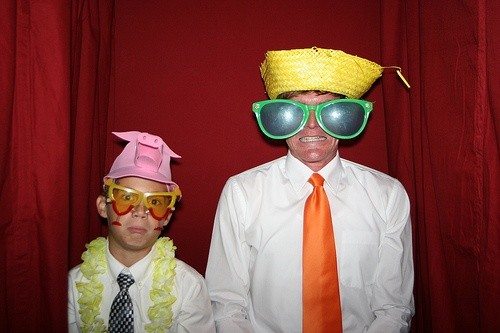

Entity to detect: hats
[259,47,410,100]
[102,131,183,191]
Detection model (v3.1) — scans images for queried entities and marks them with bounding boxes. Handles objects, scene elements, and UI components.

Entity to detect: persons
[67,156,219,333]
[206,46,418,333]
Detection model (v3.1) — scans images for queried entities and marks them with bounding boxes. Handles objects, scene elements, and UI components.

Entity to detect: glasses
[103,178,181,230]
[252,99,373,139]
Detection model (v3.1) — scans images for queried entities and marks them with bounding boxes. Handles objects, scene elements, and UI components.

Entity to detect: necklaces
[73,236,178,333]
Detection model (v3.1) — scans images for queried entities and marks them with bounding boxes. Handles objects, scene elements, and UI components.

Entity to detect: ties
[107,272,135,333]
[300,173,343,333]
